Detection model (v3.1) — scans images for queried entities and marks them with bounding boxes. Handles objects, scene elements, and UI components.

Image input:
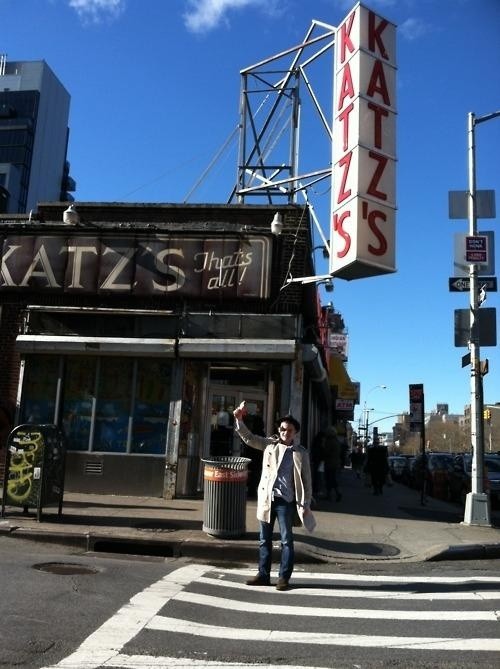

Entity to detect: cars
[388,452,500,494]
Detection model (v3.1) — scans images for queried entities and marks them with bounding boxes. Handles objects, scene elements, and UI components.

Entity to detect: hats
[277,415,300,434]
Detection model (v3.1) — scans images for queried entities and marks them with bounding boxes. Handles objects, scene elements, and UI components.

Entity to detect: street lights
[359,384,386,445]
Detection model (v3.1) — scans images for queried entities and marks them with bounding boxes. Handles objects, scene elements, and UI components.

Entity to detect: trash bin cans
[3,423,66,524]
[201,457,252,539]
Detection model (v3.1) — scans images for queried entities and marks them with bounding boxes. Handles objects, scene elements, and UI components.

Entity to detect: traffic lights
[488,410,491,420]
[483,410,487,419]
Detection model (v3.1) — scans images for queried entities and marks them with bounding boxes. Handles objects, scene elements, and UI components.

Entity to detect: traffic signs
[449,276,497,293]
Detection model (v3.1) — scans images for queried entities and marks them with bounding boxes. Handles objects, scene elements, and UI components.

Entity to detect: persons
[309,435,390,501]
[231,402,314,592]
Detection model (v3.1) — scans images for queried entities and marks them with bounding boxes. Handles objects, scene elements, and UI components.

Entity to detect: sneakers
[276,577,289,590]
[245,573,270,585]
